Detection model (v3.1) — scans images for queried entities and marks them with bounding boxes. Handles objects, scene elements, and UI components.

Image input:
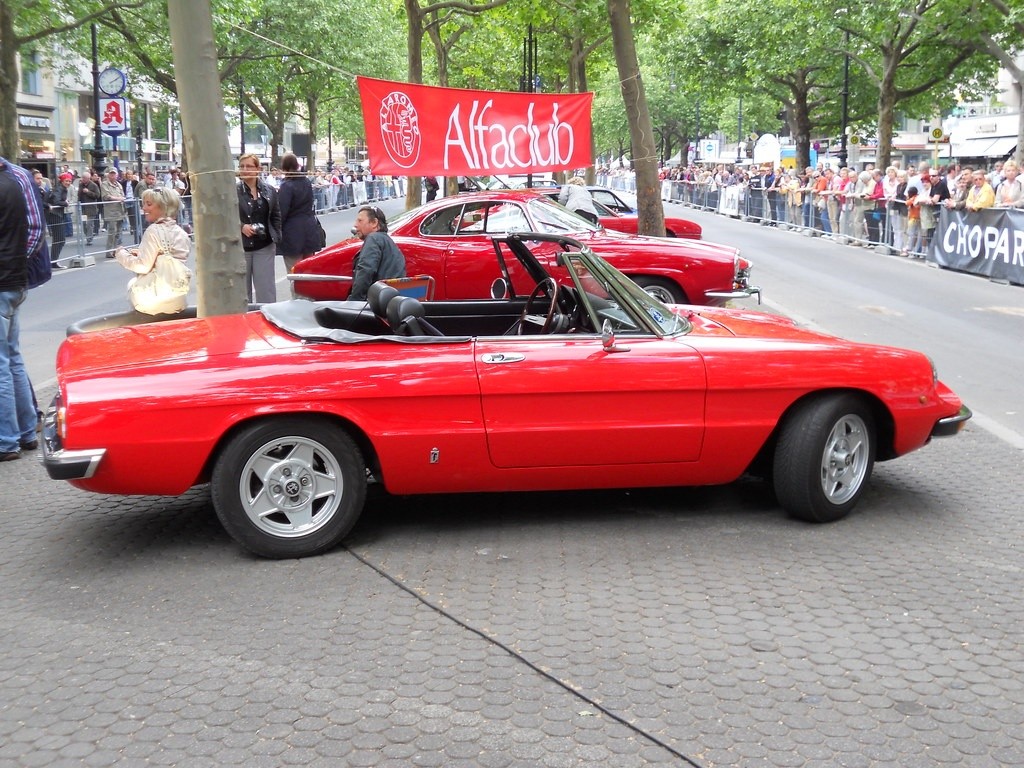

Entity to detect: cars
[455,189,703,239]
[290,189,762,308]
[516,180,558,188]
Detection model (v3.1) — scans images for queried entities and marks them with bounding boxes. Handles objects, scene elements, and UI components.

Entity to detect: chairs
[387,295,446,336]
[367,281,400,335]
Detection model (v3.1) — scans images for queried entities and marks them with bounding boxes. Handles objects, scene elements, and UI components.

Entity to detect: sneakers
[51,263,68,270]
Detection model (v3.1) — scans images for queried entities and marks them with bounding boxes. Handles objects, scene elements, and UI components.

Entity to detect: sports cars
[37,233,972,559]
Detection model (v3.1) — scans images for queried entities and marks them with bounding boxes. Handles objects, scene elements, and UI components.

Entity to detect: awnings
[937,137,1019,160]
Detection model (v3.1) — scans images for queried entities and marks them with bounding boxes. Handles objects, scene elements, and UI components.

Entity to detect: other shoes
[821,234,862,246]
[747,218,760,223]
[0,452,24,461]
[21,441,38,450]
[105,253,116,258]
[86,240,94,246]
[900,250,928,259]
[768,222,778,227]
[790,226,804,232]
[863,243,880,249]
[730,215,740,219]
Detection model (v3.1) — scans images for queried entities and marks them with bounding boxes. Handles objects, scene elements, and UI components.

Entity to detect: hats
[758,167,767,172]
[108,167,118,173]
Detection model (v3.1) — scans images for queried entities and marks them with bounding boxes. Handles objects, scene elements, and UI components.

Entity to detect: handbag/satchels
[872,211,882,222]
[315,216,327,248]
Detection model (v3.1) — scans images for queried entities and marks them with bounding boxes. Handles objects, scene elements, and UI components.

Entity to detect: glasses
[238,164,259,169]
[813,175,819,179]
[929,174,937,178]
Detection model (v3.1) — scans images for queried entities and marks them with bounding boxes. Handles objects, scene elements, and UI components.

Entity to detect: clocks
[99,68,126,96]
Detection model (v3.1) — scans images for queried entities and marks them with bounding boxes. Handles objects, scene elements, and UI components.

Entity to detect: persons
[557,176,599,228]
[30,159,411,271]
[0,156,53,462]
[425,175,439,204]
[577,157,1024,289]
[232,153,282,304]
[273,154,326,279]
[343,205,406,302]
[116,185,193,314]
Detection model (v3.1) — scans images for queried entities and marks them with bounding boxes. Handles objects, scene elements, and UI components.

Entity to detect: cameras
[251,223,267,240]
